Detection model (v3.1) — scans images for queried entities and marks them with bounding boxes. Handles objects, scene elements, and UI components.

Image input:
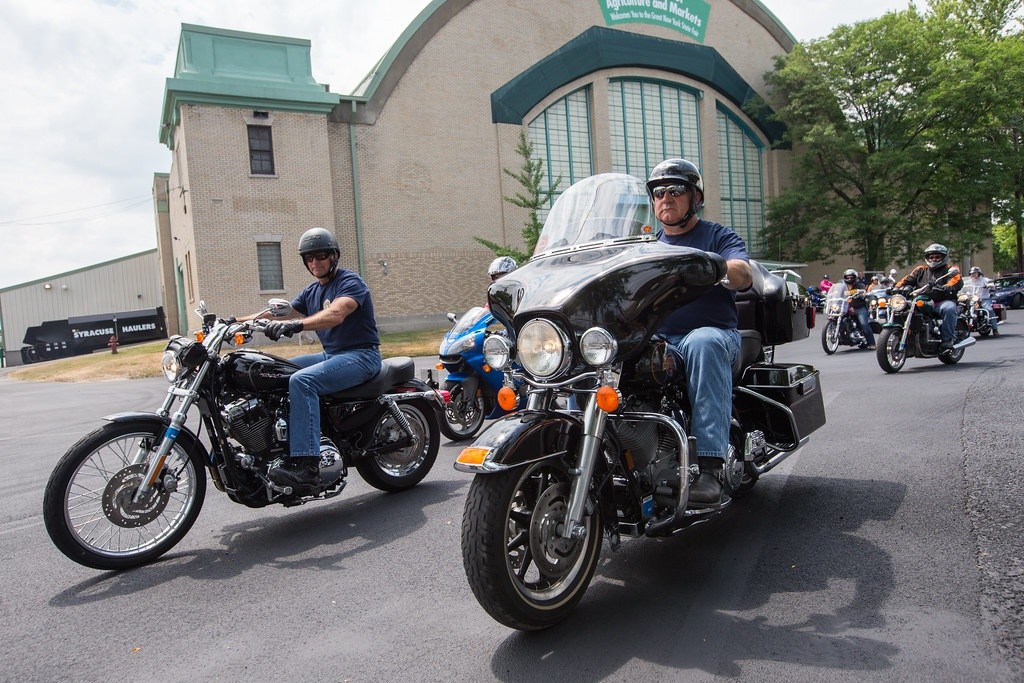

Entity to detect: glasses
[928,256,940,259]
[653,184,689,197]
[303,250,333,262]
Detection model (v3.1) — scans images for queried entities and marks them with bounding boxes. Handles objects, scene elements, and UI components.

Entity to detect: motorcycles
[43,300,452,571]
[807,285,827,314]
[865,285,893,324]
[957,277,1007,337]
[453,172,826,632]
[876,267,979,374]
[437,306,530,440]
[822,282,882,355]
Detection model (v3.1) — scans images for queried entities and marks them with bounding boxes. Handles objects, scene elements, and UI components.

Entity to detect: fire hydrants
[107,334,120,355]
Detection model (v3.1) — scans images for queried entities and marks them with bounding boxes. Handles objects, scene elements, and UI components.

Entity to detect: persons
[820,243,998,356]
[211,228,381,490]
[486,257,521,310]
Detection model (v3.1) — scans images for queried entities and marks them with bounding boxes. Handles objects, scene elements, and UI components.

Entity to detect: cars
[989,274,1024,309]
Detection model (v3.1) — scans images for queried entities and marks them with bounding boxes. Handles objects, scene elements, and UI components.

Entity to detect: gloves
[219,315,236,326]
[941,285,952,293]
[263,320,304,341]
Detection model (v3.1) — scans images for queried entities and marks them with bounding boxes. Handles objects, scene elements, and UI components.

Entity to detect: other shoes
[941,338,954,348]
[869,345,876,349]
[993,329,999,336]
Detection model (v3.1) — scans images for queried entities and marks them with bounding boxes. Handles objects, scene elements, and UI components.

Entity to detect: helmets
[924,243,949,268]
[646,158,705,206]
[871,276,878,282]
[488,257,518,280]
[890,269,898,277]
[844,269,859,285]
[969,267,984,276]
[298,227,340,260]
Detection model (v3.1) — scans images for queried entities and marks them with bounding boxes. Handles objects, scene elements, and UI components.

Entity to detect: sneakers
[269,456,322,495]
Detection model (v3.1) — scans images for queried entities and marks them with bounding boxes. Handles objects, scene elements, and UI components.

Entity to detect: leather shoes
[677,455,725,508]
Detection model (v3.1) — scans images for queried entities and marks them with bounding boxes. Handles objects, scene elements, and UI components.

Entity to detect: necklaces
[645,157,754,508]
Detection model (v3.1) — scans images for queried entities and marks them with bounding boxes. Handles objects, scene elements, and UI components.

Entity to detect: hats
[823,275,830,279]
